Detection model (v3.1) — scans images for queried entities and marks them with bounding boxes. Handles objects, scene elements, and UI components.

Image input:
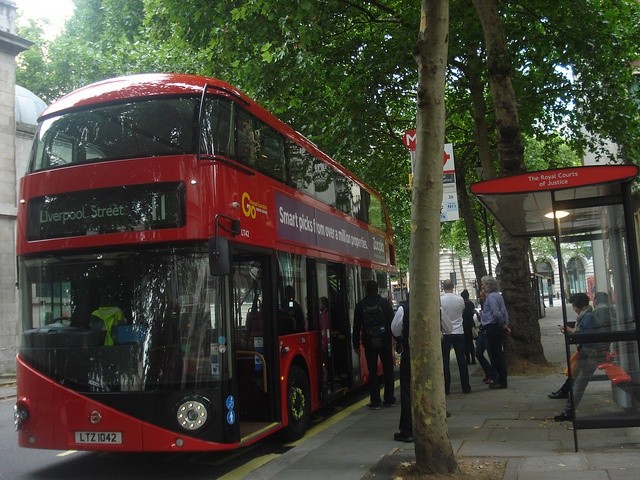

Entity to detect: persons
[352,280,397,410]
[478,275,512,390]
[439,279,471,394]
[554,293,605,422]
[548,292,611,400]
[475,284,493,384]
[390,301,415,442]
[315,295,330,370]
[460,289,476,364]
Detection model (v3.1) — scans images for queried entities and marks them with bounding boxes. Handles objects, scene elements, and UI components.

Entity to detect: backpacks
[361,303,392,352]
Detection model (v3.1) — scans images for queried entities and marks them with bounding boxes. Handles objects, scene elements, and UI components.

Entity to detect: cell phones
[556,323,562,329]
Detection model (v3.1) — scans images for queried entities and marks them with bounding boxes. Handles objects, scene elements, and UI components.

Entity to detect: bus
[13,73,397,452]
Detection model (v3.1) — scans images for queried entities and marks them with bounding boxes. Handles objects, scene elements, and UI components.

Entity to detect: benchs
[604,366,632,386]
[598,357,615,370]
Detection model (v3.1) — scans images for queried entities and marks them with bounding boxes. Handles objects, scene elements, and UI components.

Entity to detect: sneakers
[548,390,569,399]
[381,400,396,407]
[554,412,571,421]
[367,403,381,410]
[394,432,414,443]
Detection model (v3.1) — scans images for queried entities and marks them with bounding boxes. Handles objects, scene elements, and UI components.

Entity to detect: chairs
[115,322,151,351]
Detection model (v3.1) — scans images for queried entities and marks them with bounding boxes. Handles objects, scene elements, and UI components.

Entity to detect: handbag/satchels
[563,350,581,379]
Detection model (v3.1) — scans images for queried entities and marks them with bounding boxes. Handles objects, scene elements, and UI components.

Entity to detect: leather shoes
[489,381,507,389]
